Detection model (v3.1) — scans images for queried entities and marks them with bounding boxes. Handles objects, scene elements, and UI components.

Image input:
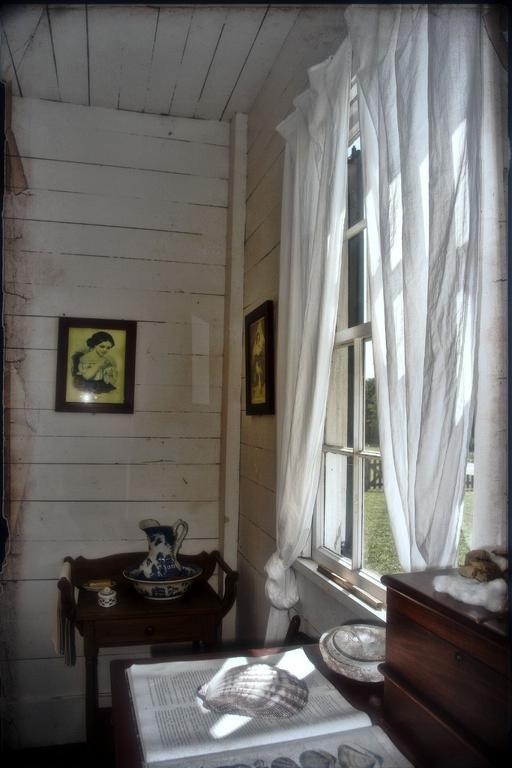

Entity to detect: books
[124,646,417,768]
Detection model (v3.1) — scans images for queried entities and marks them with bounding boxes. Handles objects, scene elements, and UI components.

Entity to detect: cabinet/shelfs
[59,549,238,767]
[376,564,512,768]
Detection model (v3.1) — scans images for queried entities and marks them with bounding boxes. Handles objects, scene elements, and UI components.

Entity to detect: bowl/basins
[122,563,202,600]
[333,622,387,663]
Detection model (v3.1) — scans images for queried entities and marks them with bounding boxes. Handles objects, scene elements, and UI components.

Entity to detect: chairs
[282,615,319,646]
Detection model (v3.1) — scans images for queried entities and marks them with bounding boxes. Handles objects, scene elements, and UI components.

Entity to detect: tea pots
[137,516,189,579]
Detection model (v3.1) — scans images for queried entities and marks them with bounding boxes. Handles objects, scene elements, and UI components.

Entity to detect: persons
[251,330,265,399]
[70,330,120,393]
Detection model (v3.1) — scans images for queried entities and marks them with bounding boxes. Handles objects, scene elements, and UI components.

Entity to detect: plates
[97,599,116,607]
[318,627,385,685]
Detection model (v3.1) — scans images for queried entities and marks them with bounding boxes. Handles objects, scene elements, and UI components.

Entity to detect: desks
[110,637,432,766]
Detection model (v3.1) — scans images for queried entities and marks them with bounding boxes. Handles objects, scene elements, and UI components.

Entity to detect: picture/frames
[55,315,136,413]
[244,299,276,414]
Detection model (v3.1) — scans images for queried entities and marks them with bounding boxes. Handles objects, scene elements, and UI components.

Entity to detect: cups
[98,589,116,602]
